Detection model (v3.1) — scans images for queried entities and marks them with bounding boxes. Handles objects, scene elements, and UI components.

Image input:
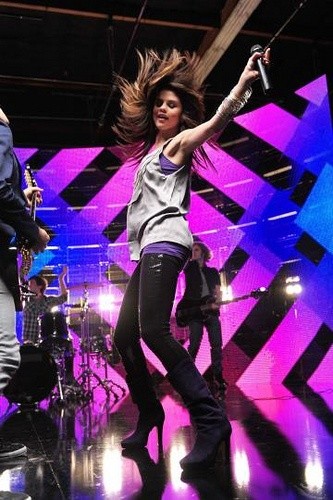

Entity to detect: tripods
[54,283,126,404]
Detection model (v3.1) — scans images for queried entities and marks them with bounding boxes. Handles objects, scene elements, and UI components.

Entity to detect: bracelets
[215,84,253,122]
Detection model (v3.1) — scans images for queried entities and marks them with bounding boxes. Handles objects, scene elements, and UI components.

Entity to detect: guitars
[175,286,272,328]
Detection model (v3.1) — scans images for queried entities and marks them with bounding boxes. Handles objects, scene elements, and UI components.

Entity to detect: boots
[121,369,165,448]
[166,356,232,472]
[211,365,227,390]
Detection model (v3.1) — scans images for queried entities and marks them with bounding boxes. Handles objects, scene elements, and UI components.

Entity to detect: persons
[175,241,228,390]
[111,46,268,469]
[0,103,71,500]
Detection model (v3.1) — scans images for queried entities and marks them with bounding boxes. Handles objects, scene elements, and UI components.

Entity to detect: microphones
[250,44,272,97]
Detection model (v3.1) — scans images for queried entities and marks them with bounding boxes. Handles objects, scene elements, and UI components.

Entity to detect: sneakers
[0,438,27,460]
